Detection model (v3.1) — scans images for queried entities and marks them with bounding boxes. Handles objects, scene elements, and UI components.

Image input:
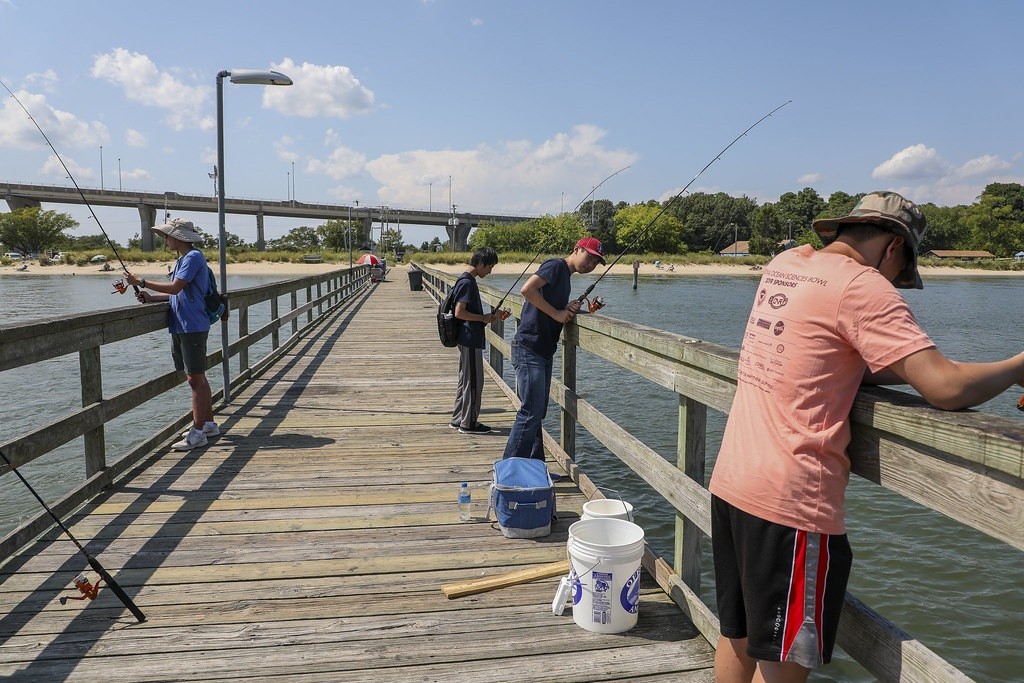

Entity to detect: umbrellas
[358,253,382,274]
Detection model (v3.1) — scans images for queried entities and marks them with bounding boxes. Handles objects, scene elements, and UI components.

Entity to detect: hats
[152,217,205,243]
[575,237,606,267]
[811,191,929,290]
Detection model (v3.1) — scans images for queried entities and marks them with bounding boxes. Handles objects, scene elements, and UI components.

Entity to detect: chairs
[372,268,391,283]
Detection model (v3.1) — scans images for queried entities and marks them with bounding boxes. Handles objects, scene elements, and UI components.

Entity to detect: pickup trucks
[282,200,303,204]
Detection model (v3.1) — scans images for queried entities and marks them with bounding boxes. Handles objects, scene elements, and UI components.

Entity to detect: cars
[3,252,23,260]
[50,255,61,265]
[163,191,179,195]
[27,253,40,260]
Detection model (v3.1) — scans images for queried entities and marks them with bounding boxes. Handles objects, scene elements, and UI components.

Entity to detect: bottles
[457,482,471,521]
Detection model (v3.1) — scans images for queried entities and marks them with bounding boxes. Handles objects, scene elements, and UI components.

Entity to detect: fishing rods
[0,451,146,621]
[0,80,147,306]
[481,165,632,329]
[575,99,793,313]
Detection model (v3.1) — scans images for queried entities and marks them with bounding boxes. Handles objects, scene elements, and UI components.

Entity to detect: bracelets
[140,278,145,289]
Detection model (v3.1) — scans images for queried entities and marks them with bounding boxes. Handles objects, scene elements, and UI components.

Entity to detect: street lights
[349,207,354,296]
[429,183,433,213]
[561,192,565,214]
[592,185,595,223]
[215,67,295,403]
[449,175,452,213]
[370,211,401,261]
[292,162,294,201]
[287,172,290,201]
[118,158,122,191]
[100,145,103,189]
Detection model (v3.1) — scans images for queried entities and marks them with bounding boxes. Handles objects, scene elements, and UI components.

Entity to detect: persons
[123,220,220,452]
[502,238,607,483]
[449,247,512,434]
[706,195,1024,683]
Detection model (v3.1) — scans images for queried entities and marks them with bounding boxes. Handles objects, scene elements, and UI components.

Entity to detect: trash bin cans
[407,269,425,291]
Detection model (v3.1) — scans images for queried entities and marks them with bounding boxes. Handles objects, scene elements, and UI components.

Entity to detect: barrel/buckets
[579,486,635,523]
[566,518,645,635]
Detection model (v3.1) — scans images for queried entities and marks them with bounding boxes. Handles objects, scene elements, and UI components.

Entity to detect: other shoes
[449,422,492,434]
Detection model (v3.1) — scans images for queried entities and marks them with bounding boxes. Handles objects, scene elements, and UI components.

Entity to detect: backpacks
[436,275,474,347]
[178,249,226,325]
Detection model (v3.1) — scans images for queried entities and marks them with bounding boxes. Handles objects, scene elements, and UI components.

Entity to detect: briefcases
[487,457,559,539]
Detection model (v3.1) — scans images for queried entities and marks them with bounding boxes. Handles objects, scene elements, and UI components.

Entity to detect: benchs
[303,254,325,263]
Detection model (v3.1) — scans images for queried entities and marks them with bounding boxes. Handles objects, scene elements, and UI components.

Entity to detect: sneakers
[182,421,220,437]
[171,426,208,450]
